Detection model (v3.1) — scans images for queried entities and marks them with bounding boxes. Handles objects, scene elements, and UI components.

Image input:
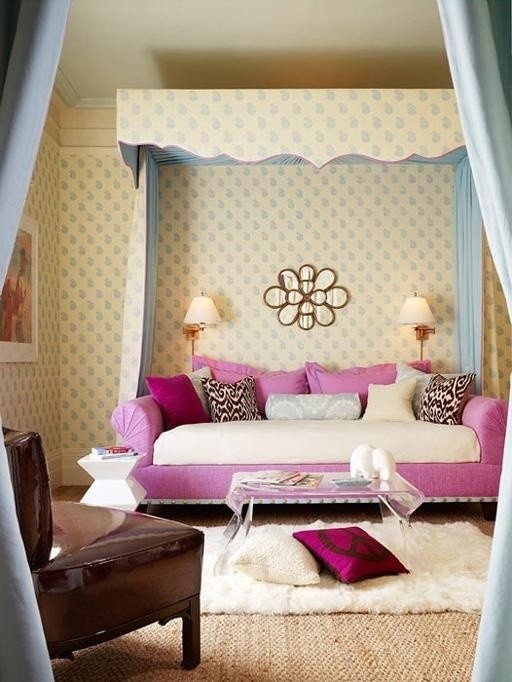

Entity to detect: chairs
[0,425,211,680]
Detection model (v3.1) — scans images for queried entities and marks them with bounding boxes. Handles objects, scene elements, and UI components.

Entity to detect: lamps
[179,294,223,373]
[399,295,438,361]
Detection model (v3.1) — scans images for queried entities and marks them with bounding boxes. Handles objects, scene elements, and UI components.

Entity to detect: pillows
[226,521,414,587]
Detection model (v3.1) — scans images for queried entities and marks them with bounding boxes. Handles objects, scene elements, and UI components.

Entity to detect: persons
[1,247,29,345]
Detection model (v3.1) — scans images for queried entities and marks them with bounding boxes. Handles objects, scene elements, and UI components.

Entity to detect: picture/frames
[0,212,42,363]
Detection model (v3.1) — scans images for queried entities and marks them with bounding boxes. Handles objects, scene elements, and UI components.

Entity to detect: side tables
[75,451,148,514]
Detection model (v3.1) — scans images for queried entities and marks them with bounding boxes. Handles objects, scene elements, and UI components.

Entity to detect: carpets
[187,521,492,623]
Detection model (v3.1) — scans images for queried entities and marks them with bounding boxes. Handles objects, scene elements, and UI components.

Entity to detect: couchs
[109,362,511,523]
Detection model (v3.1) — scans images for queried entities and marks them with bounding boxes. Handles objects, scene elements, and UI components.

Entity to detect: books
[296,473,323,489]
[242,467,300,483]
[277,472,309,485]
[90,445,133,455]
[99,451,138,460]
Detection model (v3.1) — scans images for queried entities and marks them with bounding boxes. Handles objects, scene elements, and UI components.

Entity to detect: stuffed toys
[347,442,396,481]
